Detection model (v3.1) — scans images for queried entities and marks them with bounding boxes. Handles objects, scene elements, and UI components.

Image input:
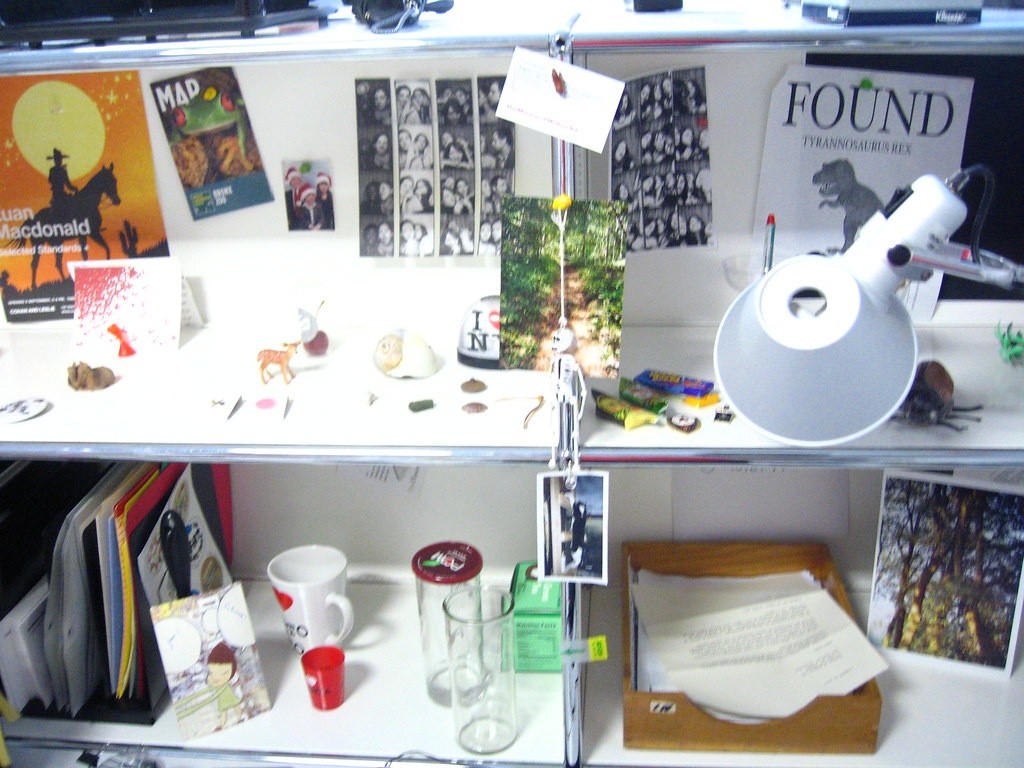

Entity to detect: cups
[442,586,519,754]
[267,544,353,654]
[301,647,344,711]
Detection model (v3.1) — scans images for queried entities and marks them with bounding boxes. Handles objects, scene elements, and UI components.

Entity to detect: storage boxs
[621,540,883,754]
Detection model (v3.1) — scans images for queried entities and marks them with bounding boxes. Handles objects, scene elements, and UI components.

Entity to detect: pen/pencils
[760,214,775,275]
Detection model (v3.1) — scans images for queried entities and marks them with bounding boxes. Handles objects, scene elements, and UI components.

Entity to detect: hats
[285,167,299,185]
[316,172,331,188]
[46,148,68,160]
[296,183,317,208]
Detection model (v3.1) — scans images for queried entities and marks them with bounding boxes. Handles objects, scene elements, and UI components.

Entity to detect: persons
[566,502,587,574]
[613,74,712,252]
[285,167,334,230]
[356,77,514,255]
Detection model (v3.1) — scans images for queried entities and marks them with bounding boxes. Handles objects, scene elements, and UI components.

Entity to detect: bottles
[412,541,485,705]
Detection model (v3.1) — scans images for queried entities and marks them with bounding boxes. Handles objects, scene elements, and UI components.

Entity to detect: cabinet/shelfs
[0,0,1024,768]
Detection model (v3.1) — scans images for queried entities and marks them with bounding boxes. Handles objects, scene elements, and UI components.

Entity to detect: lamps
[712,167,1023,449]
